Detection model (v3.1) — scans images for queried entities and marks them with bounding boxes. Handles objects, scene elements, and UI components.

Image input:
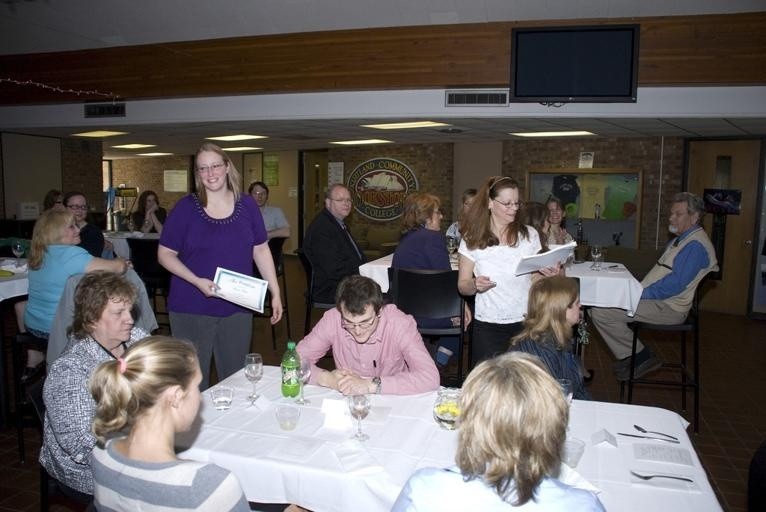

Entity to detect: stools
[14,335,49,464]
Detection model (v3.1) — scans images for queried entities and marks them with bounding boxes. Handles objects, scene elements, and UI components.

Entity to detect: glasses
[340,311,376,329]
[66,205,88,210]
[197,162,225,173]
[493,198,523,207]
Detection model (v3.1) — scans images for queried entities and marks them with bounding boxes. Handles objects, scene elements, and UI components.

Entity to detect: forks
[630,469,693,483]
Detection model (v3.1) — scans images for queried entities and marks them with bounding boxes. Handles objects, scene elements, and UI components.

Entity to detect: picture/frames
[525,168,643,251]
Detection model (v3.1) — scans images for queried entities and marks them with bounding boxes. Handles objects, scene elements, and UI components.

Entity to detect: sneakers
[613,354,658,381]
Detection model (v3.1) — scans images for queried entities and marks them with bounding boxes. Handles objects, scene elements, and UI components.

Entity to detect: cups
[560,438,585,469]
[275,406,302,431]
[210,386,234,411]
[432,387,464,432]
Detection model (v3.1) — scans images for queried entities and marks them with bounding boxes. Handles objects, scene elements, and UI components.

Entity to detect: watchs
[372,376,381,395]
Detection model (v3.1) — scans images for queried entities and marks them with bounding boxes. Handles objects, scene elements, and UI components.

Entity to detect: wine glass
[11,239,25,268]
[142,219,154,237]
[447,235,461,262]
[126,221,135,234]
[349,389,371,442]
[244,352,264,401]
[591,247,603,272]
[291,359,311,405]
[556,378,572,406]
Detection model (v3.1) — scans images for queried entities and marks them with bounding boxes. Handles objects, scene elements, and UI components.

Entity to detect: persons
[392,188,575,373]
[457,175,561,370]
[390,351,607,512]
[586,192,720,381]
[158,143,284,392]
[38,270,152,508]
[280,275,440,396]
[304,184,369,305]
[43,189,105,257]
[88,335,310,512]
[506,274,592,401]
[132,190,167,235]
[16,205,132,382]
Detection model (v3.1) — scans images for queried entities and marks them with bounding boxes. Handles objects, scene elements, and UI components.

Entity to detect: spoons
[634,424,678,440]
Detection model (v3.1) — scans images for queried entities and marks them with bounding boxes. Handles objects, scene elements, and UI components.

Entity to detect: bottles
[576,222,583,245]
[280,341,302,397]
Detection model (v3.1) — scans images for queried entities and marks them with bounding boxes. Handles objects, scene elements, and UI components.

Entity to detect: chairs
[22,359,92,510]
[561,279,583,367]
[295,248,339,359]
[77,228,104,262]
[124,237,172,338]
[124,239,176,338]
[253,238,296,350]
[386,267,474,388]
[620,261,716,434]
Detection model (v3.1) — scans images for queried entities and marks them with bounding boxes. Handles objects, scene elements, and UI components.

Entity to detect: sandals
[20,361,46,385]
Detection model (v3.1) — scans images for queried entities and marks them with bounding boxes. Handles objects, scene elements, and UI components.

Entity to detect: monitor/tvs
[508,23,641,105]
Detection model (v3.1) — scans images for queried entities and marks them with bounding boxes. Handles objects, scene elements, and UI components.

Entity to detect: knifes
[617,432,680,444]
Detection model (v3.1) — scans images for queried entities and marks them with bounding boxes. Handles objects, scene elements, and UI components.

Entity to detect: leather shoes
[583,370,593,382]
